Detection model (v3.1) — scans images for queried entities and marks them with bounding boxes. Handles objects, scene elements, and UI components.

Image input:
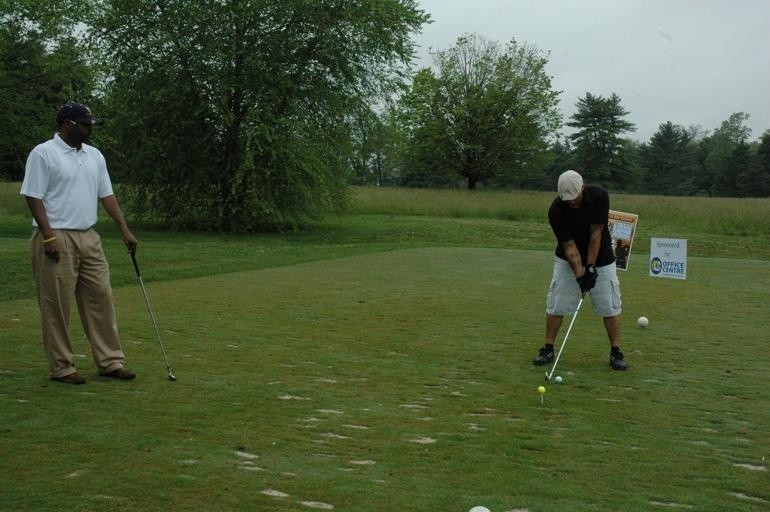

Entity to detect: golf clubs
[129,247,176,381]
[545,287,586,381]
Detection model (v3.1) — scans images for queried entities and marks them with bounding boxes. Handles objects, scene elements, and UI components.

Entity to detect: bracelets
[40,236,56,246]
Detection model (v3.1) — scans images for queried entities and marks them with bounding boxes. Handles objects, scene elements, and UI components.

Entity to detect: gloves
[584,263,598,284]
[576,273,592,291]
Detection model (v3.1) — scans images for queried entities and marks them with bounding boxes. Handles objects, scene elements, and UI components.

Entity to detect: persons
[20,103,138,385]
[615,239,630,269]
[533,170,627,370]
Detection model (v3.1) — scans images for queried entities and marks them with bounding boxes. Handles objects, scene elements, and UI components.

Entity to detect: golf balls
[555,376,562,384]
[538,386,546,394]
[637,317,649,327]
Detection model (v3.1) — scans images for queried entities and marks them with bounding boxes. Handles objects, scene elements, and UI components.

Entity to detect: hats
[55,103,104,128]
[557,169,584,202]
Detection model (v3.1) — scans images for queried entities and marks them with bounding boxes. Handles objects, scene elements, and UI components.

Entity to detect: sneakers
[100,367,136,380]
[534,343,554,365]
[51,372,86,385]
[610,351,627,370]
